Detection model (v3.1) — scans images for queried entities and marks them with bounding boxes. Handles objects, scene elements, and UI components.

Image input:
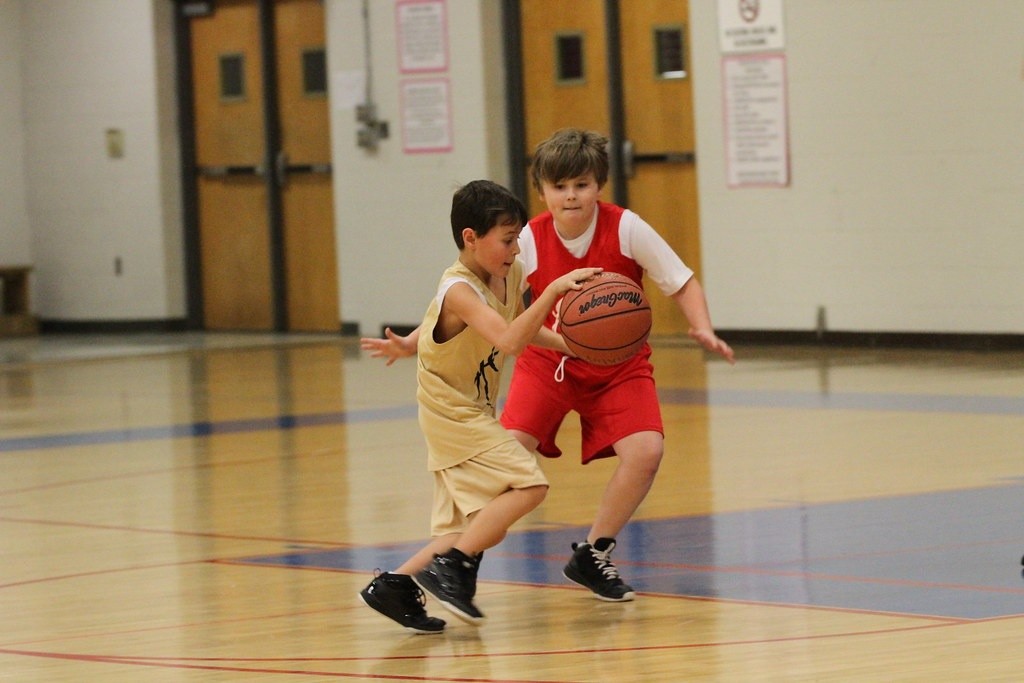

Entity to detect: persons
[360,127,738,603]
[358,181,603,634]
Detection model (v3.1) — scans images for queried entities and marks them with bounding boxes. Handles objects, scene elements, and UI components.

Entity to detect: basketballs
[557,270,656,365]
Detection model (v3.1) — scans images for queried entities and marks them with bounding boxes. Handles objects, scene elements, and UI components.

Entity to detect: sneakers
[410,546,488,625]
[357,568,447,635]
[561,536,636,602]
